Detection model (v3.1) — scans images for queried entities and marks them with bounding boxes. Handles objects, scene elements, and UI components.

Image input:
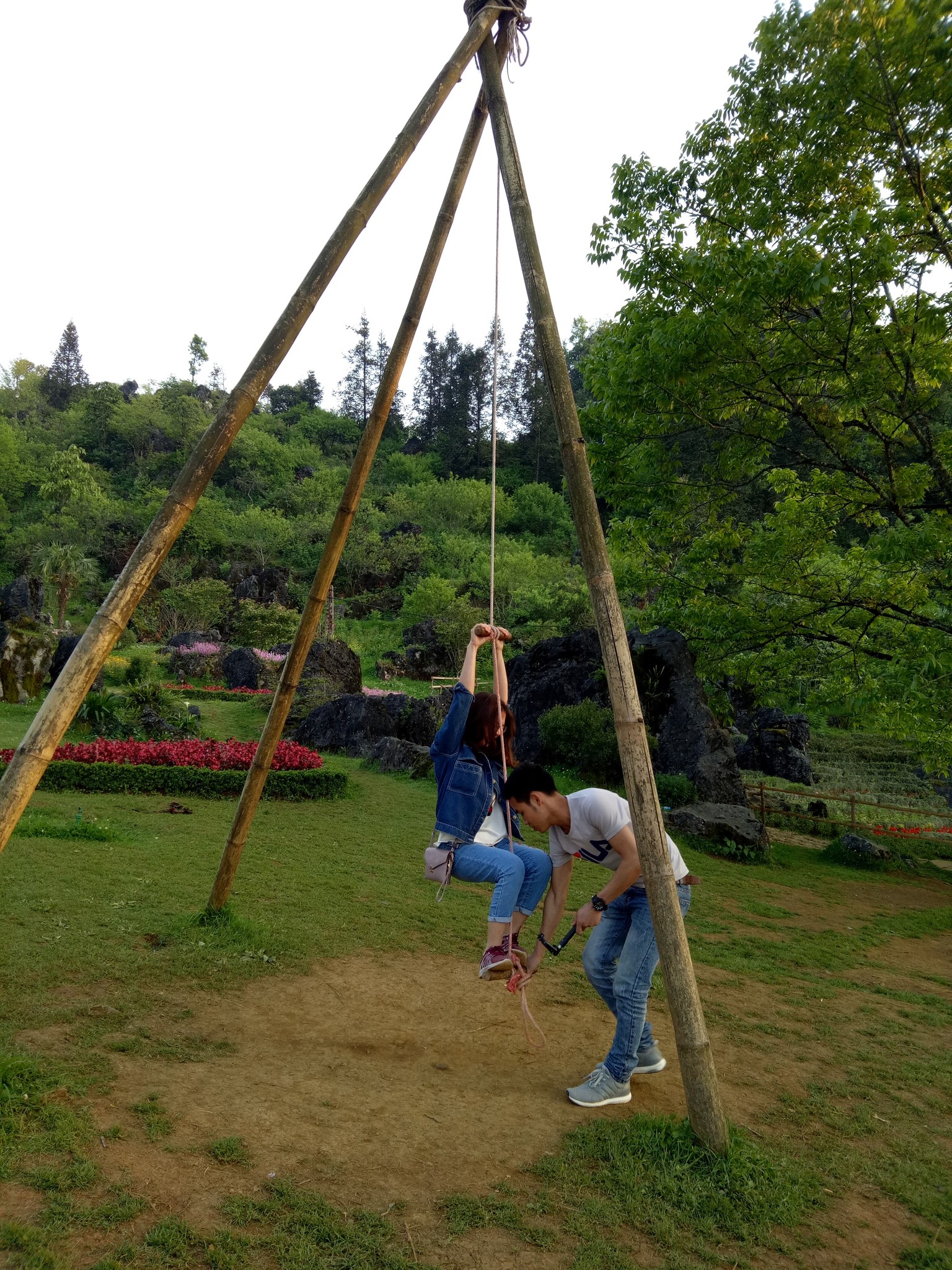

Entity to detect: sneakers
[478,947,514,979]
[596,1040,667,1074]
[565,1065,633,1107]
[502,932,527,965]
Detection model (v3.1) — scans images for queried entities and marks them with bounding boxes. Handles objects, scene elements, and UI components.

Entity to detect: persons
[499,761,701,1107]
[429,623,554,979]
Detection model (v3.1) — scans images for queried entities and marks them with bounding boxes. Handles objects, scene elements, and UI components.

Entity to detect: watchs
[591,894,608,912]
